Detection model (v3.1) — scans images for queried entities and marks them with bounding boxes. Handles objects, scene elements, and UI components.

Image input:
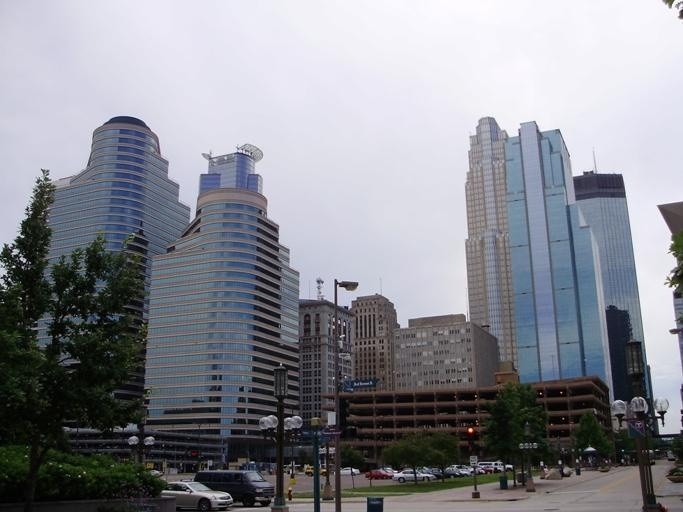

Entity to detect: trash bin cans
[576,469,580,475]
[367,496,383,512]
[500,477,507,489]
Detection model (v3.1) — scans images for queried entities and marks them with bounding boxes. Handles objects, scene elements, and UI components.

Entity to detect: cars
[334,467,361,475]
[365,462,513,483]
[161,481,233,512]
[668,455,675,461]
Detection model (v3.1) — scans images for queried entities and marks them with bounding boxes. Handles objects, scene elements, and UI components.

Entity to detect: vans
[195,470,275,506]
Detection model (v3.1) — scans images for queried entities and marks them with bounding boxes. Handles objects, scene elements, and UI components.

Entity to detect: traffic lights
[467,426,475,435]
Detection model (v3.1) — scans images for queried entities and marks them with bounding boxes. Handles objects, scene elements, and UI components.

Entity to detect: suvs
[305,466,327,477]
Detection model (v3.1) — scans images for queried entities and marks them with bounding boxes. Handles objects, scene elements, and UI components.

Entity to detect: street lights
[259,367,303,512]
[128,436,155,463]
[612,396,669,512]
[335,279,358,512]
[519,421,538,492]
